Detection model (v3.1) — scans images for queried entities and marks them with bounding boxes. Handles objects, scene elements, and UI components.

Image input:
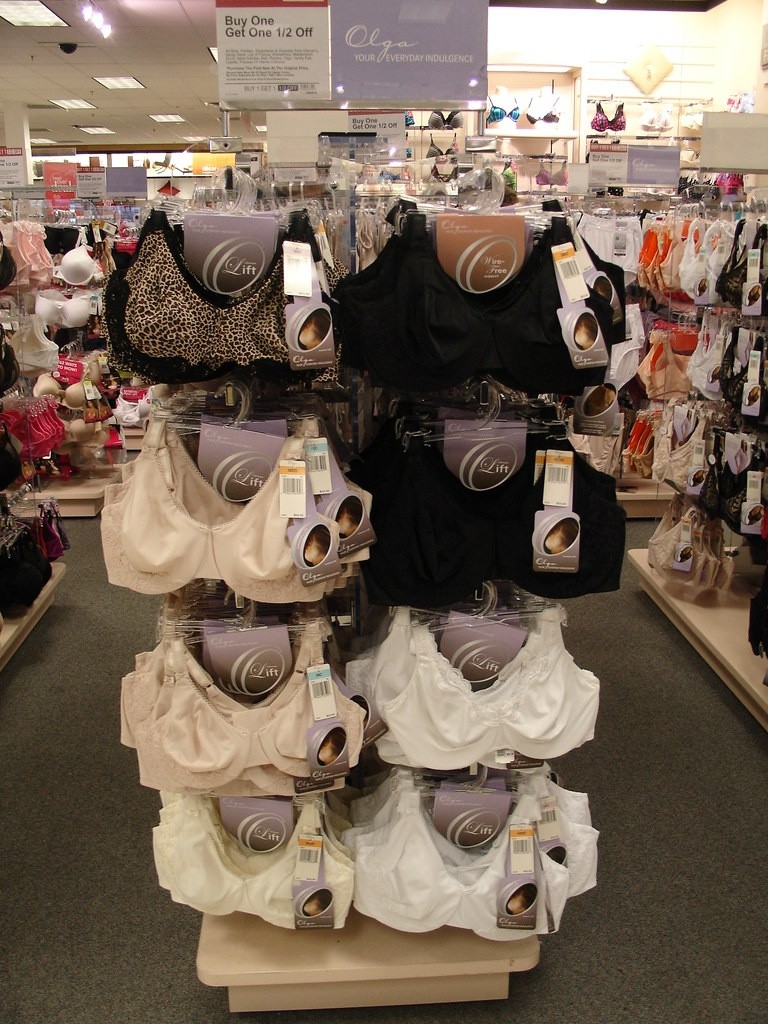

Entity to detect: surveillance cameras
[58,43,77,54]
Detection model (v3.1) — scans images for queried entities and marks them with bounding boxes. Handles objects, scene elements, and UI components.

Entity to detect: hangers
[0,167,768,813]
